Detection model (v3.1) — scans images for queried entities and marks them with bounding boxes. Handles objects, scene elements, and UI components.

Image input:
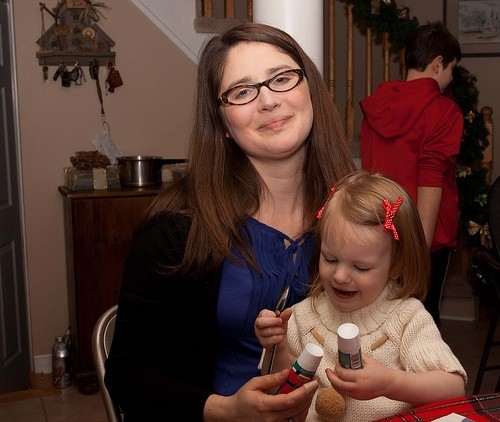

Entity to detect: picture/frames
[441,0,500,58]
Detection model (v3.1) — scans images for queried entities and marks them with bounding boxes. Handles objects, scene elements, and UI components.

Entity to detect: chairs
[455,179,500,397]
[90,305,121,422]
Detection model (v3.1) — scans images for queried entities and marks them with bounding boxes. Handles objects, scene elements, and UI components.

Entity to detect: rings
[288,418,292,422]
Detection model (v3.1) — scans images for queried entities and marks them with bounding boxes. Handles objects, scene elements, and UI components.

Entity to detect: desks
[58,179,173,366]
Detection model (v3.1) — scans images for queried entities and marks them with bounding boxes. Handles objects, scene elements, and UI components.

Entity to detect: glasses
[215,68,305,110]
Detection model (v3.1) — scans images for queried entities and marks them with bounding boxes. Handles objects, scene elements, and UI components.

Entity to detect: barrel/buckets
[116,156,160,187]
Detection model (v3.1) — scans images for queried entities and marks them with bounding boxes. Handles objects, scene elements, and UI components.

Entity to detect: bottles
[53,336,71,389]
[337,323,363,369]
[275,342,324,395]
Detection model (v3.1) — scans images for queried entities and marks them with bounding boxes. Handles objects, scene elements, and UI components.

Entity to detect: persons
[358,20,463,326]
[103,23,360,422]
[255,169,449,422]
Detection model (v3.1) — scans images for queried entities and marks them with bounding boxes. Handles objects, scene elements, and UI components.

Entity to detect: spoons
[269,284,290,376]
